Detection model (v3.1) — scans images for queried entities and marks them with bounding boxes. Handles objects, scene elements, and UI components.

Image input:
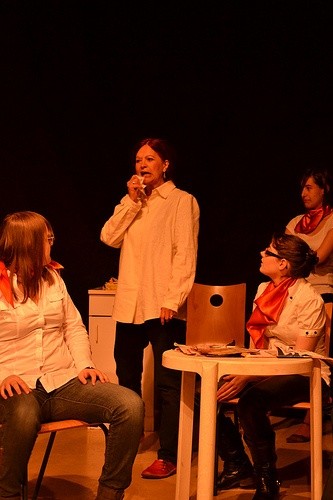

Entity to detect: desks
[161,348,325,500]
[87,288,157,432]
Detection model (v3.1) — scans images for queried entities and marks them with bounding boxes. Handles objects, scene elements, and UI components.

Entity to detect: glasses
[265,248,282,260]
[48,234,55,241]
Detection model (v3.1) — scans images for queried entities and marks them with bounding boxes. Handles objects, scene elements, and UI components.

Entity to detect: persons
[100,138,200,480]
[215,233,326,500]
[283,168,333,302]
[0,211,145,500]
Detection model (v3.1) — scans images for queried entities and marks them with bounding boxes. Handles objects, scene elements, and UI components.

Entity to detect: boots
[218,453,253,490]
[252,463,279,500]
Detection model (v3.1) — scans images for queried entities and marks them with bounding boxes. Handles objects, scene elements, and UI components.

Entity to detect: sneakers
[141,459,177,478]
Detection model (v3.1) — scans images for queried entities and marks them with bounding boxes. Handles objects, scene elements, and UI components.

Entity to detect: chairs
[185,283,333,500]
[19,418,108,500]
